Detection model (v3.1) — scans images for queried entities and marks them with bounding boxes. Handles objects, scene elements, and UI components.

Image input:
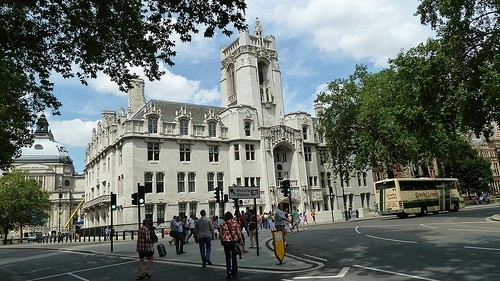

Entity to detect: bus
[374,177,465,219]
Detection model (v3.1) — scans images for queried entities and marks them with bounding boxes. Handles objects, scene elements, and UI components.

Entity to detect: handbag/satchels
[170,230,177,237]
[235,242,243,255]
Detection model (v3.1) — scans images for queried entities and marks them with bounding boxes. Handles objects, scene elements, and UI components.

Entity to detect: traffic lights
[138,186,145,204]
[224,194,228,203]
[132,193,138,205]
[330,192,334,199]
[111,194,116,210]
[214,187,220,203]
[280,180,290,197]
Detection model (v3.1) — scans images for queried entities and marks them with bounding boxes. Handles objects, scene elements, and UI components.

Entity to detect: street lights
[58,185,63,231]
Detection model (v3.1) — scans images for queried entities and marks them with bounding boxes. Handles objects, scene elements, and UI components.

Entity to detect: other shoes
[227,271,231,279]
[203,261,206,268]
[205,259,211,264]
[143,272,152,278]
[177,251,186,255]
[136,276,143,279]
[169,241,172,246]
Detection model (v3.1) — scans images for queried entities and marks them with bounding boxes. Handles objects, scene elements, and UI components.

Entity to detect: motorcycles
[472,195,489,204]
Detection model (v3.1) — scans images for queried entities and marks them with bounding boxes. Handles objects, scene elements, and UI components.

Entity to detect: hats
[143,220,151,223]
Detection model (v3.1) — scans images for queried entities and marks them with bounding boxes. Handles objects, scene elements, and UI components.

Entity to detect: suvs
[28,232,39,242]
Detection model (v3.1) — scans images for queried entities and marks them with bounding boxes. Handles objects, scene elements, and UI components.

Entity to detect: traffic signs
[229,186,260,199]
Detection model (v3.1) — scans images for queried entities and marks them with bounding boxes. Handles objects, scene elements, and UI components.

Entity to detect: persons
[472,191,490,205]
[136,217,158,281]
[344,207,358,221]
[219,212,244,281]
[167,202,292,255]
[104,225,115,241]
[194,210,214,268]
[286,205,318,232]
[373,202,378,214]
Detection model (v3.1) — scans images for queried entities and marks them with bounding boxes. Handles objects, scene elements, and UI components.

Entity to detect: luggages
[155,240,167,257]
